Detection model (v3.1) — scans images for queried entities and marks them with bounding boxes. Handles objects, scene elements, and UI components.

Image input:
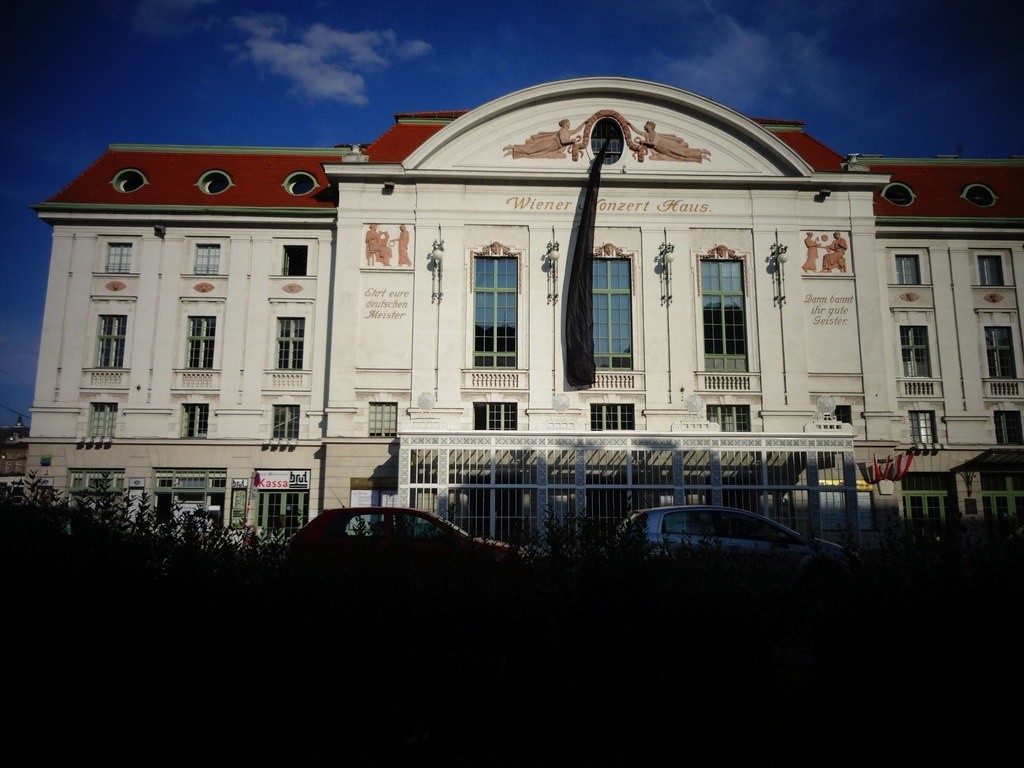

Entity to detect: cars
[618,504,846,574]
[286,506,532,575]
